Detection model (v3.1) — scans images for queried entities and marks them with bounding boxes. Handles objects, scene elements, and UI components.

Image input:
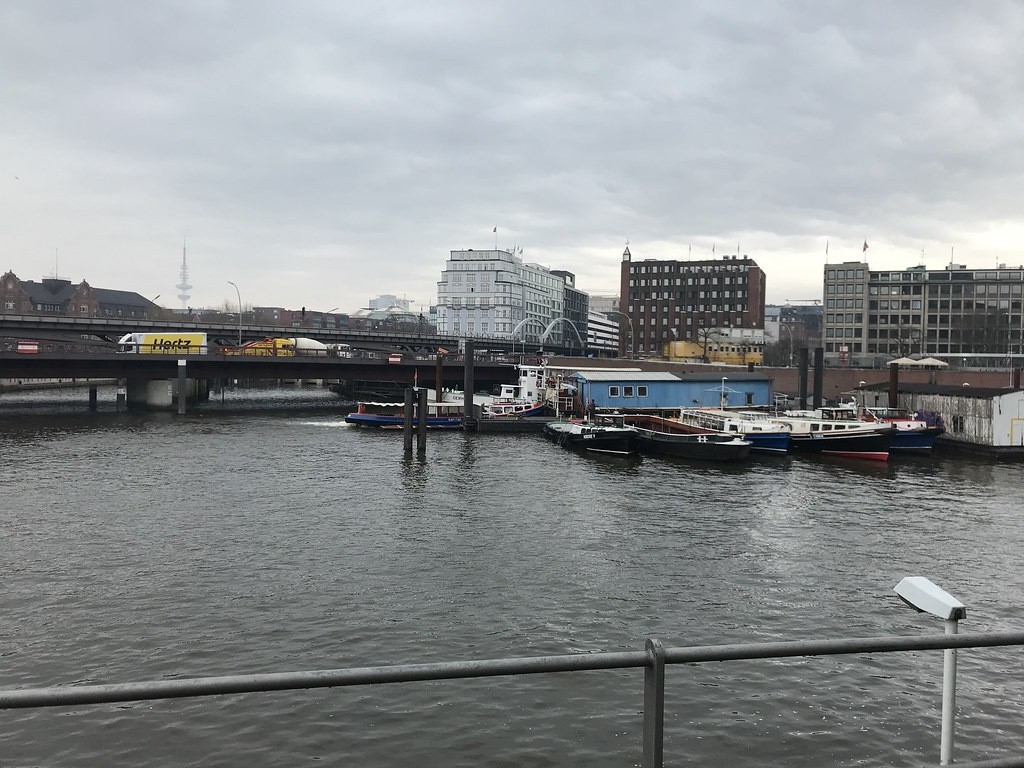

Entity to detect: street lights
[225,279,243,350]
[891,575,968,768]
[776,320,794,368]
[143,294,162,318]
[609,310,635,360]
[317,307,341,335]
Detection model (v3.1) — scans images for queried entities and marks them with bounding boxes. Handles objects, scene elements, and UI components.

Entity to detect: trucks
[117,332,208,355]
[215,337,296,358]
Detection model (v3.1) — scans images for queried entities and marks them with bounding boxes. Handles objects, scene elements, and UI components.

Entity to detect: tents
[887,357,948,367]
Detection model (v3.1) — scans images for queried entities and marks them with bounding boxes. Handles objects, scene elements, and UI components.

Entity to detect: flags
[493,227,497,232]
[863,241,869,252]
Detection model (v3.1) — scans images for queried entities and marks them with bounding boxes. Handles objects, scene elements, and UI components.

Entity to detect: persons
[588,399,596,419]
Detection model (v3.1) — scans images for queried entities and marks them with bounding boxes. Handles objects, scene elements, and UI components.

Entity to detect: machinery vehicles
[287,337,353,358]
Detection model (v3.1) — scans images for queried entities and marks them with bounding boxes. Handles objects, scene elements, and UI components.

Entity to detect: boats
[343,380,948,466]
